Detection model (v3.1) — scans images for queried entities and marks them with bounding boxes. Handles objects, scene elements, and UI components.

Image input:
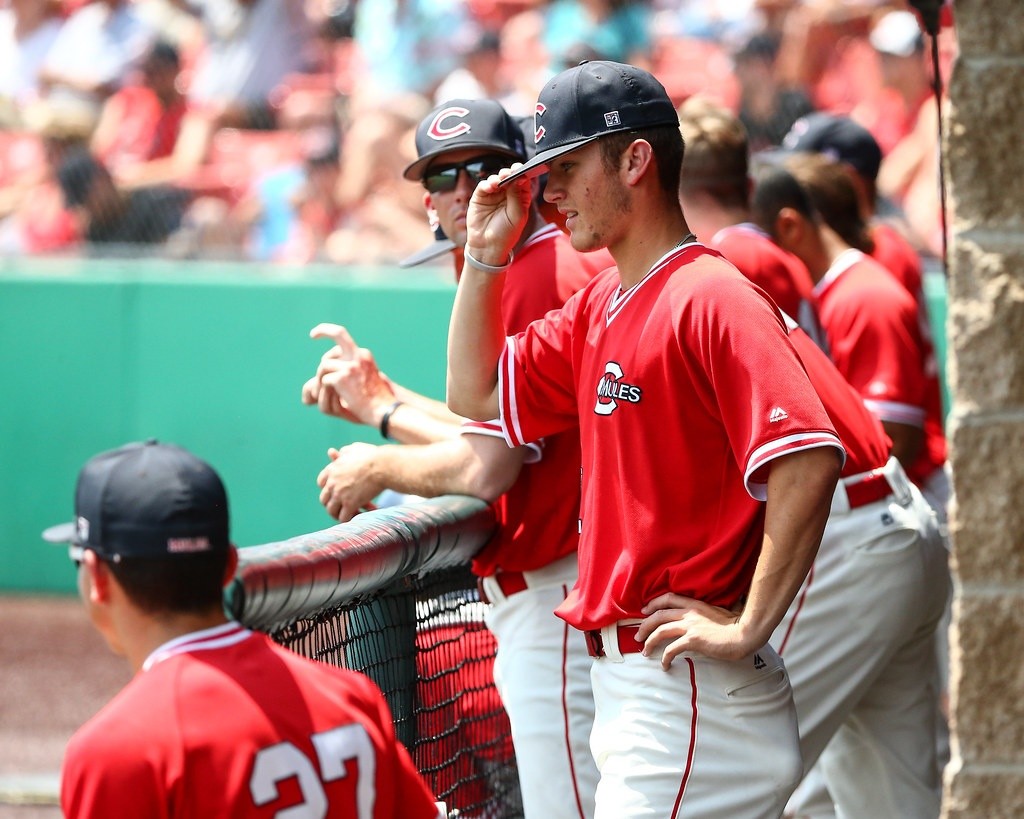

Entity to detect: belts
[846,474,890,508]
[476,570,526,602]
[584,624,645,658]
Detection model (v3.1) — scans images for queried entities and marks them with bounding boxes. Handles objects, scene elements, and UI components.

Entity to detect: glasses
[420,154,511,194]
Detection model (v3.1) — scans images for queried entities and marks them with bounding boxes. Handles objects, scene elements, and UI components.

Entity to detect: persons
[42,440,442,819]
[303,60,957,819]
[0,0,958,267]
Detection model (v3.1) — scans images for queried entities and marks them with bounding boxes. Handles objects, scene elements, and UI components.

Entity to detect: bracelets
[380,402,405,438]
[463,242,513,273]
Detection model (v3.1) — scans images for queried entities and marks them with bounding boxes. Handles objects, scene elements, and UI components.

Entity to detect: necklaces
[675,233,697,248]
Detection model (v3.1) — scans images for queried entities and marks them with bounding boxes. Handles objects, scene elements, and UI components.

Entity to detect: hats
[493,59,682,188]
[55,152,93,207]
[780,110,880,180]
[397,193,459,269]
[43,438,231,562]
[402,96,527,183]
